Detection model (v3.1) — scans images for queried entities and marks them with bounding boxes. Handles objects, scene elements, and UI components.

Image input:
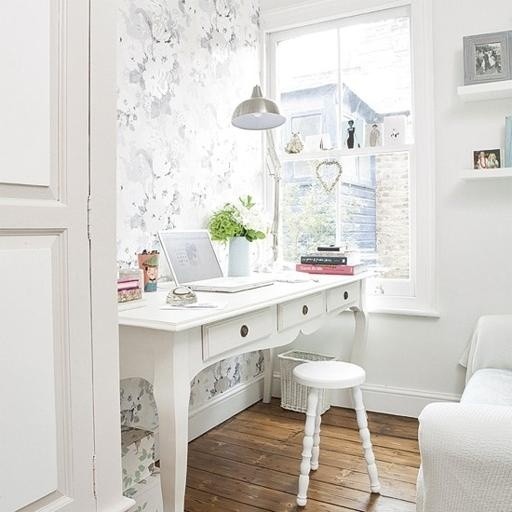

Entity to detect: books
[297,263,365,276]
[301,250,359,265]
[317,245,340,250]
[118,267,144,302]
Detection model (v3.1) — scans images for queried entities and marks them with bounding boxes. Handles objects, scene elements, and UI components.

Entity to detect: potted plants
[210,196,266,277]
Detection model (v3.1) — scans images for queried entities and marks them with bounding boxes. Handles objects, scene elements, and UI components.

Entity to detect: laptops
[156,227,277,292]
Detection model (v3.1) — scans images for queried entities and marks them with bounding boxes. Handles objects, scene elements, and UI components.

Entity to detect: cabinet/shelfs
[456,80,511,180]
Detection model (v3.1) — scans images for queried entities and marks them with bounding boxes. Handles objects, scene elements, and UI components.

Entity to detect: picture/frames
[461,30,511,85]
[472,147,504,170]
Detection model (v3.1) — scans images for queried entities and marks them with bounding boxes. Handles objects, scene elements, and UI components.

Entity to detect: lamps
[231,84,287,261]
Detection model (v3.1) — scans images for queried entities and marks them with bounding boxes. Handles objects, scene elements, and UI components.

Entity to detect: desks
[118,273,367,511]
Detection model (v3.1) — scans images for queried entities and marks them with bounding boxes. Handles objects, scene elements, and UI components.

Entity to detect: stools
[292,362,380,505]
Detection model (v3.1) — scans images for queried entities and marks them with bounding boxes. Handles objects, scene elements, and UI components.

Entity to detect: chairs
[414,314,509,510]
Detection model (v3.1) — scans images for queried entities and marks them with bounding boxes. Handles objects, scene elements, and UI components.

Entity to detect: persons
[343,117,360,147]
[479,45,500,73]
[366,120,381,146]
[487,154,497,168]
[474,150,489,168]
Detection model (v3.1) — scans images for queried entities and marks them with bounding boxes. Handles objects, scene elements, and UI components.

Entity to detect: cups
[138,253,160,292]
